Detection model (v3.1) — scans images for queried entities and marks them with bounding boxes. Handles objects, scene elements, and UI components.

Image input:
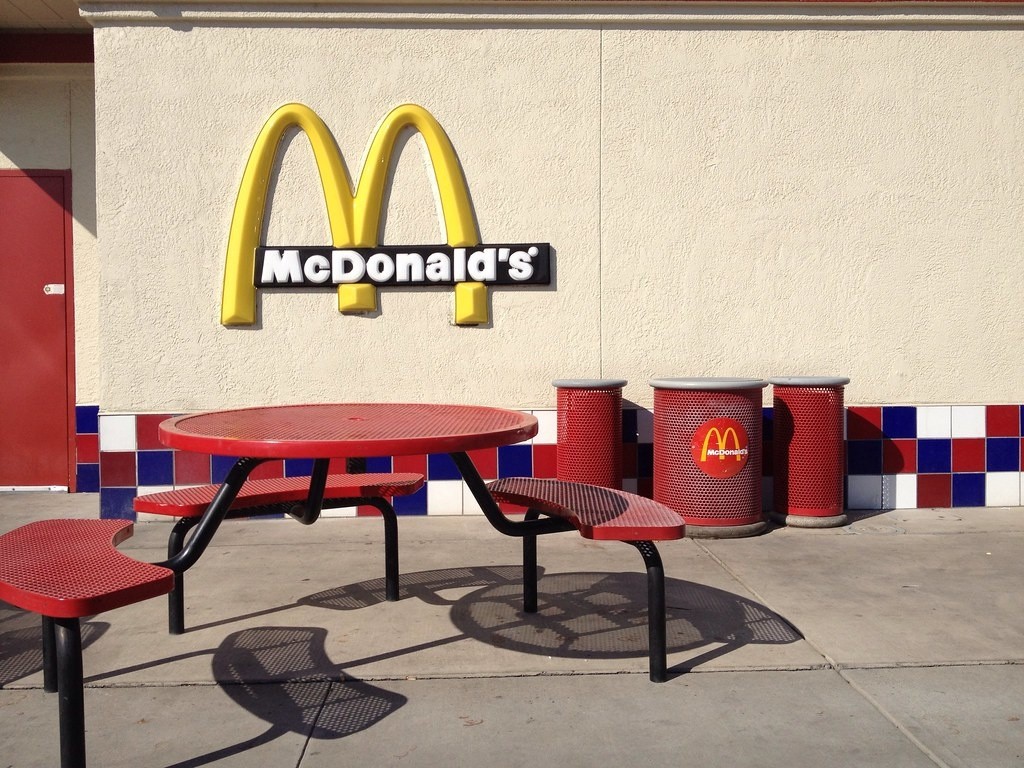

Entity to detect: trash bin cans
[648,377,772,540]
[767,375,851,528]
[551,378,626,493]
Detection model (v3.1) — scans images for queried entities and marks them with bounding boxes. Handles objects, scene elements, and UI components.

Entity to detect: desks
[152,402,575,576]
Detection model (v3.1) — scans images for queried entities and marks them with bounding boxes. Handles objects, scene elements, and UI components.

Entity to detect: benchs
[486,477,686,683]
[134,472,426,635]
[0,518,176,768]
